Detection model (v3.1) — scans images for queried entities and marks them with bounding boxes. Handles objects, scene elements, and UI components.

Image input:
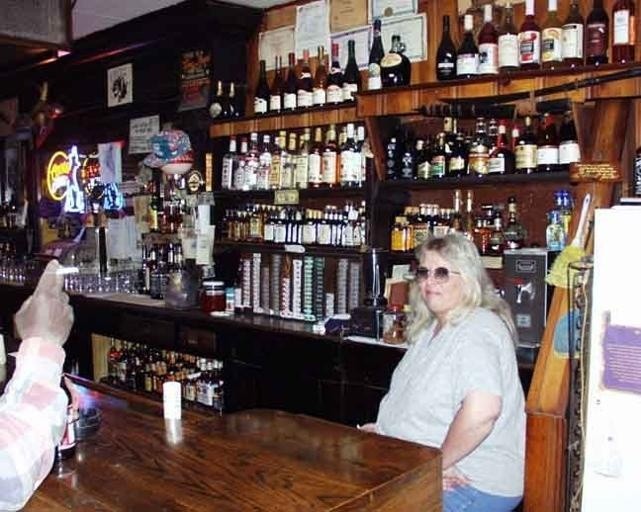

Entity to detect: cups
[0,255,133,298]
[162,381,182,420]
[163,419,183,447]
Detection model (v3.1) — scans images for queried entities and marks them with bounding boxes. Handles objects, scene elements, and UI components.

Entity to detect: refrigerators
[569,206,641,511]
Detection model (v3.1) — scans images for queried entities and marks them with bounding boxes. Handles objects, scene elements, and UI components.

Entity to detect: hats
[143,129,191,168]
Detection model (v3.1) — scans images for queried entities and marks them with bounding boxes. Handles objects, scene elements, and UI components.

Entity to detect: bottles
[283,53,299,111]
[632,146,641,197]
[435,1,638,78]
[383,303,406,344]
[313,46,328,108]
[269,55,286,115]
[381,35,412,88]
[253,60,270,116]
[134,157,230,314]
[0,189,23,255]
[344,40,362,105]
[327,44,346,106]
[298,49,314,110]
[207,78,242,123]
[367,20,386,91]
[218,102,581,262]
[105,336,224,413]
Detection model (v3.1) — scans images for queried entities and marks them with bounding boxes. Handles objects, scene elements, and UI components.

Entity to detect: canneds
[201,281,224,313]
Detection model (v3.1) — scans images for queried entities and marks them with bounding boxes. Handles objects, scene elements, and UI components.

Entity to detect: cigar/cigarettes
[52,265,78,276]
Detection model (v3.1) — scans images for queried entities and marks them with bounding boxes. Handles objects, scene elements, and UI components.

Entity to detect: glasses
[414,267,460,283]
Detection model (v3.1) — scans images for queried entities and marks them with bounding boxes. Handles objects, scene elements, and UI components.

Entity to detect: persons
[1,253,78,511]
[357,232,529,512]
